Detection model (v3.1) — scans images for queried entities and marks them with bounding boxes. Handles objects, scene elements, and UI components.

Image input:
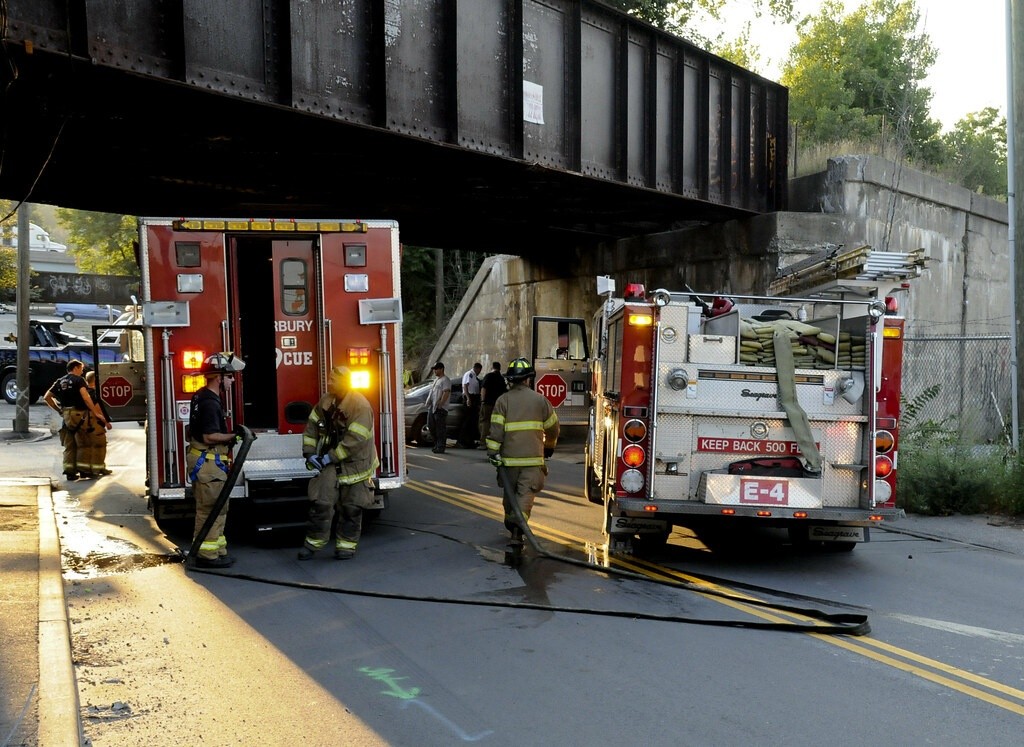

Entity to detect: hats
[431,362,444,369]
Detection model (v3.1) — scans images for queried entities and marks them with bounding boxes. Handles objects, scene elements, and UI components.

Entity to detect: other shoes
[67,475,79,480]
[196,557,232,568]
[432,448,441,453]
[80,471,93,478]
[297,546,315,560]
[100,468,112,475]
[219,554,236,563]
[335,550,353,559]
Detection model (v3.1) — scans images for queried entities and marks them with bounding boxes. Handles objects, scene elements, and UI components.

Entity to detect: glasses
[224,374,232,379]
[332,367,343,378]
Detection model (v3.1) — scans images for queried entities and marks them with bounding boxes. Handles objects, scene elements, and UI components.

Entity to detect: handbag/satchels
[57,421,66,446]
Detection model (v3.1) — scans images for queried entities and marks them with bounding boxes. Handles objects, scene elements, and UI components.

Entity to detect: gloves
[308,454,323,471]
[321,454,331,466]
[487,456,502,466]
[234,430,258,441]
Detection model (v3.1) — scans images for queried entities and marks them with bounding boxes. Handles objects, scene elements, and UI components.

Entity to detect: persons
[482,361,507,440]
[424,362,451,454]
[485,358,560,546]
[462,363,483,447]
[86,370,113,476]
[188,352,246,566]
[297,365,380,560]
[43,359,106,480]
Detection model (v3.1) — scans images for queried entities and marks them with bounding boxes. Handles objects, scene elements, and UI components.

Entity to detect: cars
[405,377,483,447]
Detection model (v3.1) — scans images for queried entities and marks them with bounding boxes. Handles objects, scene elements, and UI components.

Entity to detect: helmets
[502,357,536,383]
[199,351,246,372]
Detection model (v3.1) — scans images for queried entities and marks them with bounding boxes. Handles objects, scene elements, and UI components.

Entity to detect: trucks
[0,343,125,405]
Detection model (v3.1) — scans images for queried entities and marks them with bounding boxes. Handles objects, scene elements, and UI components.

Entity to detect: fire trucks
[528,275,905,562]
[92,217,406,531]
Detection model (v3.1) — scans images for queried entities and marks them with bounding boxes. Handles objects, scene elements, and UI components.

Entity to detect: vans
[0,222,67,253]
[54,303,120,323]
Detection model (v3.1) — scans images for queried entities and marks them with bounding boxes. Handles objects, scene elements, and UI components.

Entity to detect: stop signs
[100,376,134,408]
[535,373,568,409]
